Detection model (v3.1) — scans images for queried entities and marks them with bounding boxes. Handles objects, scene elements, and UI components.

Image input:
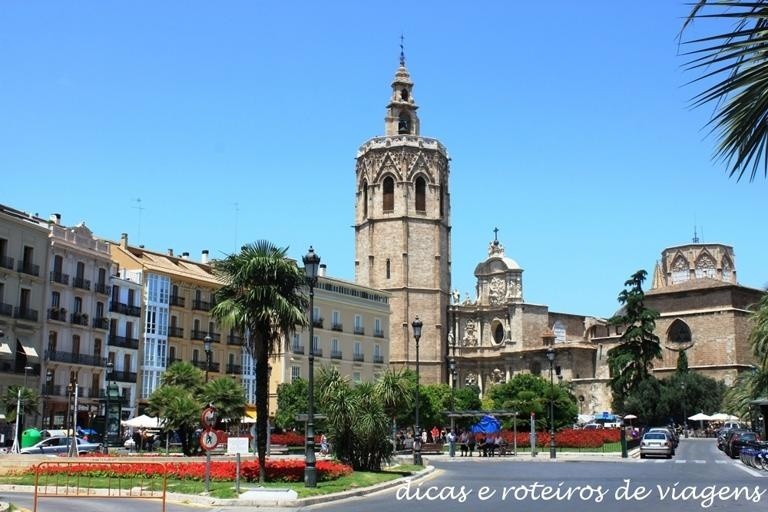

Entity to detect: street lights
[202,334,214,382]
[300,245,320,487]
[409,315,423,466]
[103,362,114,454]
[544,345,559,460]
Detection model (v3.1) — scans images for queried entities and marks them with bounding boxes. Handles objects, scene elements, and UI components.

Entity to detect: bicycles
[740,440,768,472]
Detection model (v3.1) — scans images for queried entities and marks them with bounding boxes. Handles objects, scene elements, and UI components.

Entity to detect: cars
[716,422,756,457]
[449,359,458,388]
[640,427,680,459]
[20,435,102,455]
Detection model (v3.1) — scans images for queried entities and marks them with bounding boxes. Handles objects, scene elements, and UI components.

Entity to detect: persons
[406,425,504,456]
[131,429,158,452]
[320,430,329,457]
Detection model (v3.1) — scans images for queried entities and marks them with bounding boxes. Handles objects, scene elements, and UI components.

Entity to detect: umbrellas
[687,413,739,429]
[121,413,171,450]
[624,414,638,425]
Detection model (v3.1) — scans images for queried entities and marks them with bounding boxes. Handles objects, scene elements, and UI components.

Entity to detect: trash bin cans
[21,428,41,449]
